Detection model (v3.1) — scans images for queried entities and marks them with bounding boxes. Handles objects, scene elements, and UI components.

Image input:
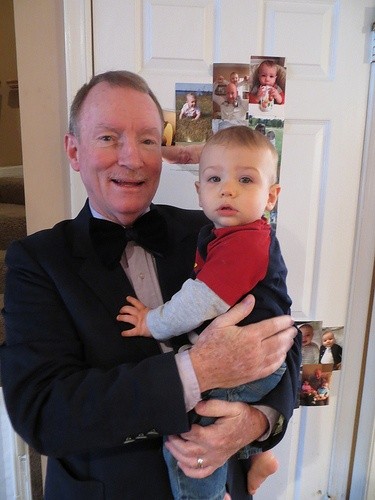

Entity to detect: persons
[318,331,342,371]
[249,61,285,104]
[302,368,330,405]
[213,72,249,121]
[178,92,200,121]
[117,125,292,500]
[299,324,319,364]
[0,71,302,500]
[254,122,277,149]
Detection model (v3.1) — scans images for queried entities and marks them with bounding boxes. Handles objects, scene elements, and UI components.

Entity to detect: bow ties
[88,206,167,272]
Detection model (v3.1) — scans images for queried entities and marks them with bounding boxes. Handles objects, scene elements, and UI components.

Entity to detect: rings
[197,458,203,469]
